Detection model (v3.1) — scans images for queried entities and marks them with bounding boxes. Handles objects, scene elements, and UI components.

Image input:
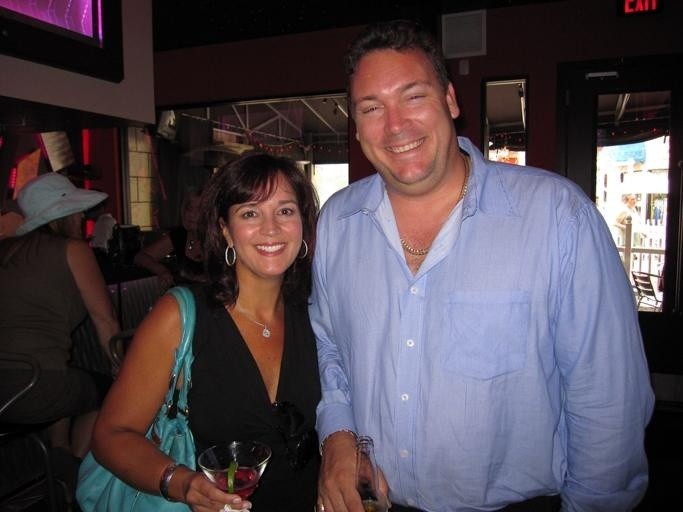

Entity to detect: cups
[197,441,272,503]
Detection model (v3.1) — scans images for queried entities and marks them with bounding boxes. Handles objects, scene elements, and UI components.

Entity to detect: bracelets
[159,461,185,503]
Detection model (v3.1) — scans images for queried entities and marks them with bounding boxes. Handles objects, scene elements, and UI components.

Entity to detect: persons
[90,154,321,512]
[307,20,658,512]
[134,187,204,287]
[1,172,123,504]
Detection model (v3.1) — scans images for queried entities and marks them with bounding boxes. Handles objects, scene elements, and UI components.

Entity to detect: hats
[16,172,109,236]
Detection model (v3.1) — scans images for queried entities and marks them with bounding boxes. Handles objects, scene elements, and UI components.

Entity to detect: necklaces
[400,156,470,256]
[230,304,285,337]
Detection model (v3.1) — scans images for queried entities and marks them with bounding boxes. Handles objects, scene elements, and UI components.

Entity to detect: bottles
[356,436,386,512]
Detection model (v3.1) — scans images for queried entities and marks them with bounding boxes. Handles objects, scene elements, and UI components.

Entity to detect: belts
[387,495,562,512]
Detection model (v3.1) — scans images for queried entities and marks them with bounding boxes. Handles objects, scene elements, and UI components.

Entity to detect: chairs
[0,351,98,510]
[631,270,663,313]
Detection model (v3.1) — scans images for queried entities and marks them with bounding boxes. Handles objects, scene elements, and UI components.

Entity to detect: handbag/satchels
[76,405,196,512]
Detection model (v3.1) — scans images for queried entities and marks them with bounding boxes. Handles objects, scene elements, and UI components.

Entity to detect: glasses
[272,401,317,459]
